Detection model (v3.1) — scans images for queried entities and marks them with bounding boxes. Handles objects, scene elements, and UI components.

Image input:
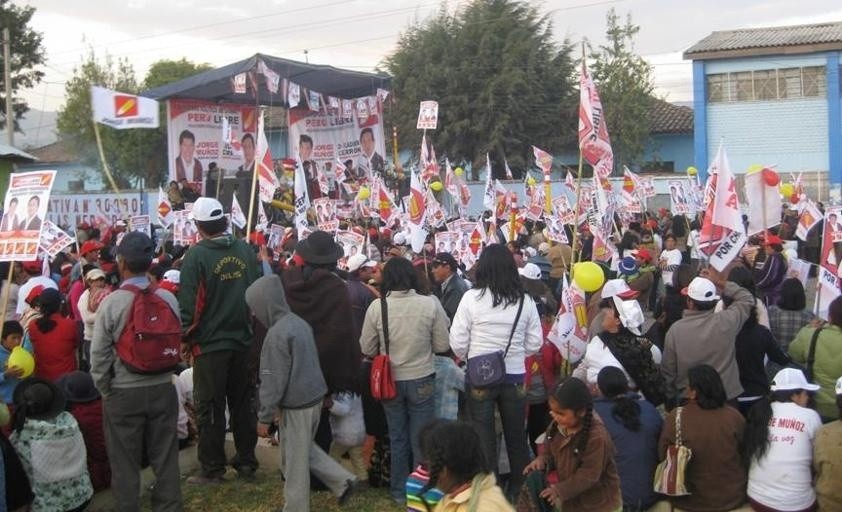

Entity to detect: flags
[91,87,161,129]
[255,113,281,203]
[699,138,748,271]
[417,56,613,181]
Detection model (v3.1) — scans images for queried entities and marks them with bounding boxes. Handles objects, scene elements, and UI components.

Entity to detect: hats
[612,296,645,337]
[188,196,224,222]
[14,377,65,419]
[769,368,821,392]
[763,235,783,246]
[86,268,106,280]
[384,248,402,257]
[116,231,154,256]
[518,242,653,275]
[80,240,105,256]
[687,277,720,302]
[517,263,542,282]
[346,252,378,274]
[21,258,43,272]
[55,370,100,402]
[600,279,641,300]
[294,230,344,265]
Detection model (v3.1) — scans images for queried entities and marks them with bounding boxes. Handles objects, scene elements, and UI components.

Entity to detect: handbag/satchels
[466,350,507,389]
[653,445,699,496]
[368,354,399,401]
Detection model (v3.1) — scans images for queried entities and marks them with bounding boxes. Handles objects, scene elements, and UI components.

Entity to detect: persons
[176,129,204,183]
[237,133,256,172]
[299,135,313,161]
[360,128,384,176]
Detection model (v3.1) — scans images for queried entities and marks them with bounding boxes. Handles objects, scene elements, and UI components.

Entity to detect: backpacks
[111,282,181,375]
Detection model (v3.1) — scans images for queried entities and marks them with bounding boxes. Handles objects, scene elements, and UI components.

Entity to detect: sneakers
[337,475,357,507]
[234,463,257,481]
[186,473,223,487]
